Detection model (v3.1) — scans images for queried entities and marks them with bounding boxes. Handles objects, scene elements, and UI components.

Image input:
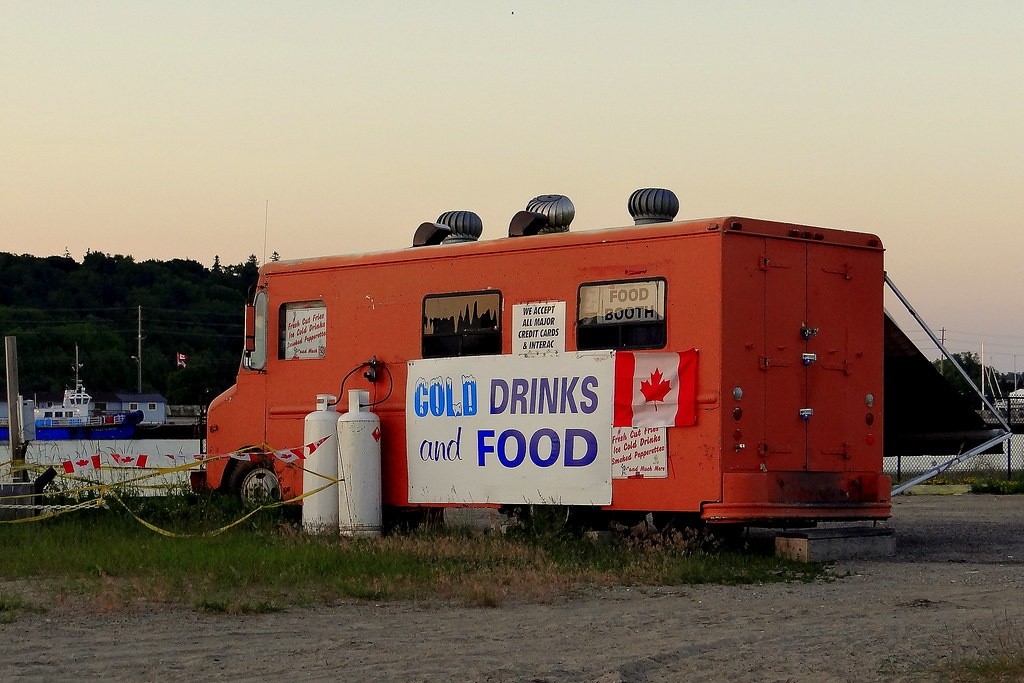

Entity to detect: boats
[0,343,145,442]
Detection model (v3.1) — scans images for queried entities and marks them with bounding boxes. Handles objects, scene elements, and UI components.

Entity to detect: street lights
[131,305,142,393]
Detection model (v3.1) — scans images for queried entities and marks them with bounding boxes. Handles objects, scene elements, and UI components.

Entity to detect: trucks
[200,216,891,530]
